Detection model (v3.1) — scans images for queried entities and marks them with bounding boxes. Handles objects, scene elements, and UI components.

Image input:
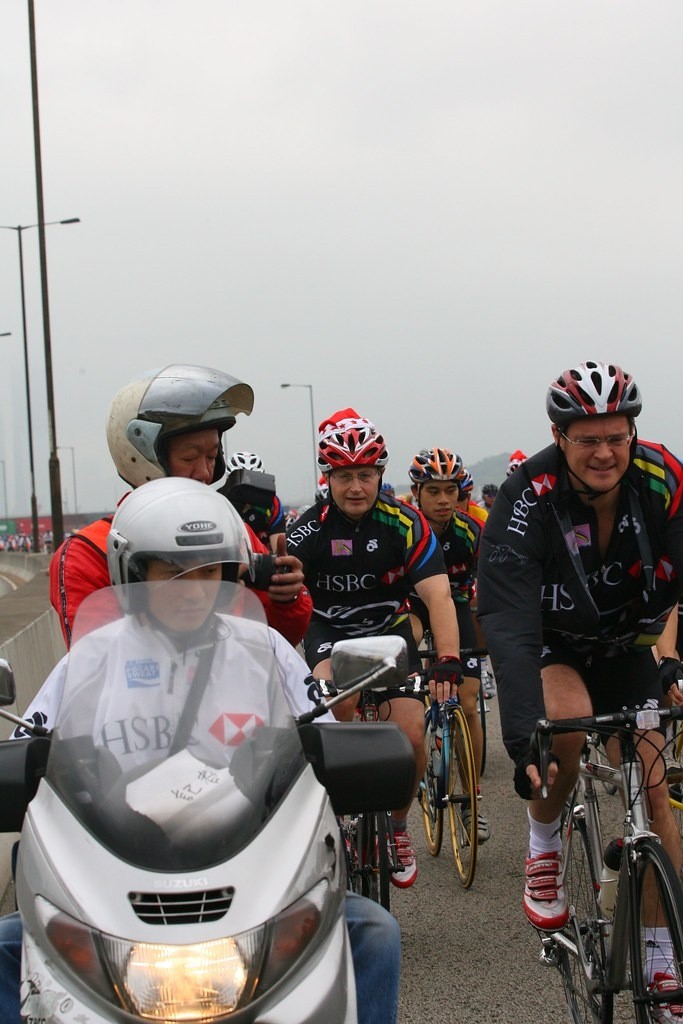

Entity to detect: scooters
[0,579,425,1023]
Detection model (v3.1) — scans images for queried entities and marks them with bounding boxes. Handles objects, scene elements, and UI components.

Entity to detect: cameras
[215,470,292,592]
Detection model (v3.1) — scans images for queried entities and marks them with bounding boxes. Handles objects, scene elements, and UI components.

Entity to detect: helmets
[482,484,499,495]
[506,464,521,478]
[546,360,642,424]
[380,483,394,493]
[106,376,236,488]
[225,449,265,480]
[317,427,389,473]
[457,468,474,491]
[408,448,466,482]
[107,476,241,614]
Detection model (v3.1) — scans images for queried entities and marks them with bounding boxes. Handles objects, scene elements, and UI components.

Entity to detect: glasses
[457,491,470,502]
[484,494,496,498]
[330,468,381,484]
[557,425,635,448]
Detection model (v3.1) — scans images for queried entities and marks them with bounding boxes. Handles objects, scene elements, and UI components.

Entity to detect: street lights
[0,218,83,557]
[279,380,320,493]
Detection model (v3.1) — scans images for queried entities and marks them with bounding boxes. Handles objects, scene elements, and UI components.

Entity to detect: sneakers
[663,749,683,783]
[648,971,683,1024]
[462,809,490,842]
[523,851,570,934]
[481,670,496,699]
[388,832,417,888]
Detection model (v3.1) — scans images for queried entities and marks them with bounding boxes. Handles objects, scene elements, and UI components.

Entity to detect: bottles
[432,742,442,777]
[598,840,624,915]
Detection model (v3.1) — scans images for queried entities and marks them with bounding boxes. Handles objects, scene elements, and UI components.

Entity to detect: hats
[317,475,328,491]
[318,407,374,444]
[508,449,528,468]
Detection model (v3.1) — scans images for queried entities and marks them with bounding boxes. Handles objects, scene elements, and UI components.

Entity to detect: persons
[286,409,466,887]
[381,450,683,848]
[0,476,400,1024]
[47,364,313,652]
[477,361,683,1024]
[0,530,72,554]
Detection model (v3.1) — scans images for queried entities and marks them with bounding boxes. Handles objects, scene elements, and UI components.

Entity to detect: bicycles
[303,674,430,913]
[529,703,682,1024]
[417,647,491,888]
